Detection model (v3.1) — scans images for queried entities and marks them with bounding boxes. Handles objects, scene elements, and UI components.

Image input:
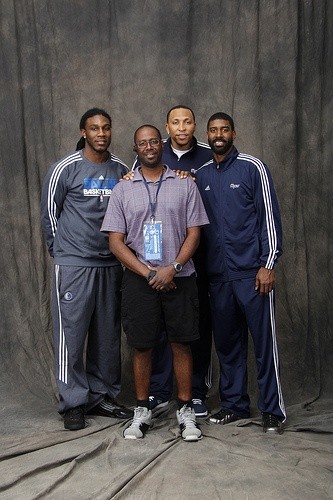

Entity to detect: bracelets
[147,270,157,283]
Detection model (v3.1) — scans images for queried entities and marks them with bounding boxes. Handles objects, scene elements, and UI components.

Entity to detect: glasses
[137,139,162,148]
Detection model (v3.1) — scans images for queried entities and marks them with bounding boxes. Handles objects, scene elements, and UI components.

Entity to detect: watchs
[171,262,183,273]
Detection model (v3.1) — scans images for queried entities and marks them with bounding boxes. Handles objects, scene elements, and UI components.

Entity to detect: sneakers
[84,399,134,419]
[191,398,208,416]
[123,406,153,440]
[64,406,85,430]
[148,395,169,411]
[208,409,246,424]
[262,412,284,435]
[175,404,203,441]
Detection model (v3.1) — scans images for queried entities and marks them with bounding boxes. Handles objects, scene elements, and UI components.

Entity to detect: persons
[101,125,210,442]
[193,111,288,434]
[118,104,212,417]
[41,107,132,430]
